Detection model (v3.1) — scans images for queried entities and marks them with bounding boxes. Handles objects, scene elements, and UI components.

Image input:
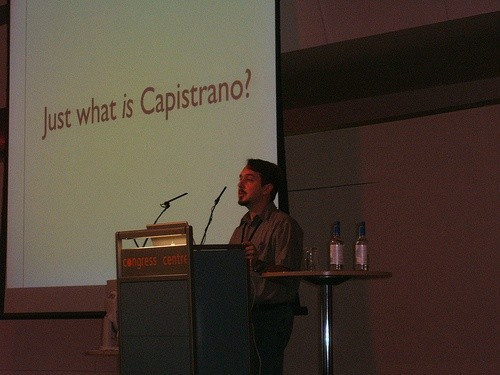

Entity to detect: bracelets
[253,262,257,271]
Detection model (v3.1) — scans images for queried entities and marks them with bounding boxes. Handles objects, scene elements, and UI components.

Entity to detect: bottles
[329,220,345,271]
[353,220,370,271]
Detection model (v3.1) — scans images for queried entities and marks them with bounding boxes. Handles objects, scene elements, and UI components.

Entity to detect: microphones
[160,193,188,205]
[215,186,227,203]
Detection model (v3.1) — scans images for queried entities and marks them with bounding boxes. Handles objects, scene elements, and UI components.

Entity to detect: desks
[261,268,393,375]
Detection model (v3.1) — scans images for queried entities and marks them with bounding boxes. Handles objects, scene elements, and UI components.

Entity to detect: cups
[304,247,319,272]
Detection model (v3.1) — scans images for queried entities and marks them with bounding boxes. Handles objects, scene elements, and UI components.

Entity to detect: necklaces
[239,220,262,244]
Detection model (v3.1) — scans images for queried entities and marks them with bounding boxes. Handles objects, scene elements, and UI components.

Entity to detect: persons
[227,159,303,375]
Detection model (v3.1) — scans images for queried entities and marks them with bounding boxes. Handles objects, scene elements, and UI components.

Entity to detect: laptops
[146,221,189,246]
